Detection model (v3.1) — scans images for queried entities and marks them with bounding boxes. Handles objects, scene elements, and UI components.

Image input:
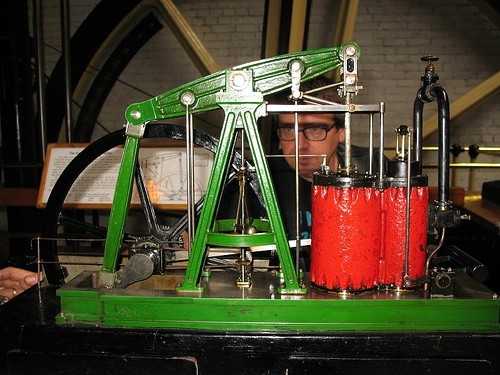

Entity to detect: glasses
[273,122,337,141]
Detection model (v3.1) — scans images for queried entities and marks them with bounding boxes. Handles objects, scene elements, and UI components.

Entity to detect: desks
[1,277,500,374]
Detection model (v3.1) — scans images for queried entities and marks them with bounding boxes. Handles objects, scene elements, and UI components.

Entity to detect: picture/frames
[36,141,201,212]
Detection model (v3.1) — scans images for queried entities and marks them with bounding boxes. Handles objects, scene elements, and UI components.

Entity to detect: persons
[0,74,391,301]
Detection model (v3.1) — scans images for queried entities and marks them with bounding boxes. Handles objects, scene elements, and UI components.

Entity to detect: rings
[13,289,17,295]
